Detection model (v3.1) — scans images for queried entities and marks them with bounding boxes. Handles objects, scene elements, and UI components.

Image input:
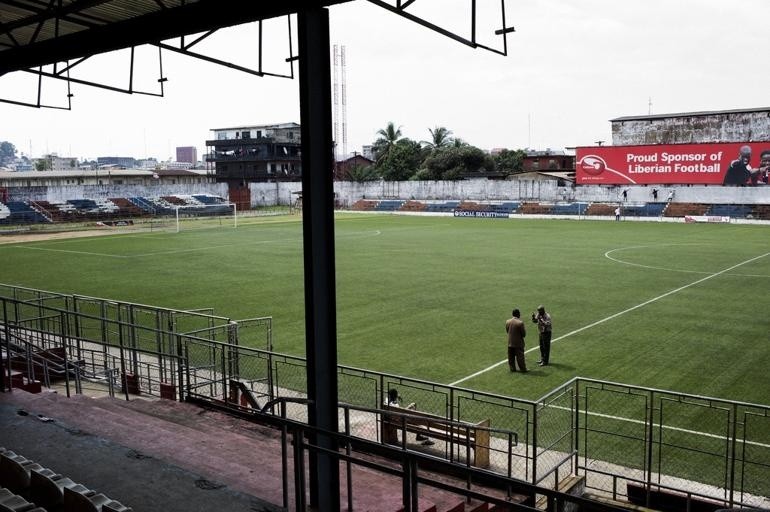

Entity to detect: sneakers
[510,366,531,373]
[415,434,436,446]
[536,359,549,367]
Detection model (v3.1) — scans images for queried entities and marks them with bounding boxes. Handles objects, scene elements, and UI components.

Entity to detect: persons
[666,190,675,202]
[614,206,621,221]
[505,308,531,373]
[294,197,301,216]
[751,149,770,185]
[531,305,552,367]
[560,188,568,201]
[620,188,630,202]
[721,144,769,188]
[384,388,437,447]
[650,188,660,201]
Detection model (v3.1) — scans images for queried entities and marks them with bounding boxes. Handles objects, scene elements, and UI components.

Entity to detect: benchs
[334,199,769,220]
[628,484,728,512]
[384,405,491,470]
[0,191,234,225]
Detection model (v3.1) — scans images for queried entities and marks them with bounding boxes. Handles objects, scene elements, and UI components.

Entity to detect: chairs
[0,446,133,511]
